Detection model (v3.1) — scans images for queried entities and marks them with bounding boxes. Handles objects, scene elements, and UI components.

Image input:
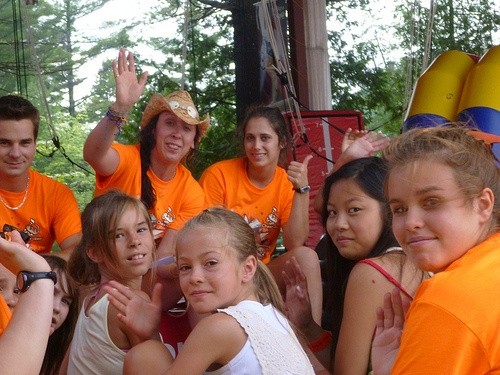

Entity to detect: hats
[141,92,210,146]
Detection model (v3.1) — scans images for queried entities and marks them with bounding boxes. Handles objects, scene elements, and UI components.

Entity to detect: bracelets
[107,107,129,136]
[305,332,333,353]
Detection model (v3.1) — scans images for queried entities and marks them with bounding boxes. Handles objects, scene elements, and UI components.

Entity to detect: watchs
[292,185,311,193]
[14,270,58,291]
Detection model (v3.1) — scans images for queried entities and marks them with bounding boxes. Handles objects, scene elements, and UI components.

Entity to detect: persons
[0,94,500,375]
[83,48,210,282]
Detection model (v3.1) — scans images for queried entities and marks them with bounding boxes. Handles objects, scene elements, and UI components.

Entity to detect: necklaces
[0,176,30,210]
